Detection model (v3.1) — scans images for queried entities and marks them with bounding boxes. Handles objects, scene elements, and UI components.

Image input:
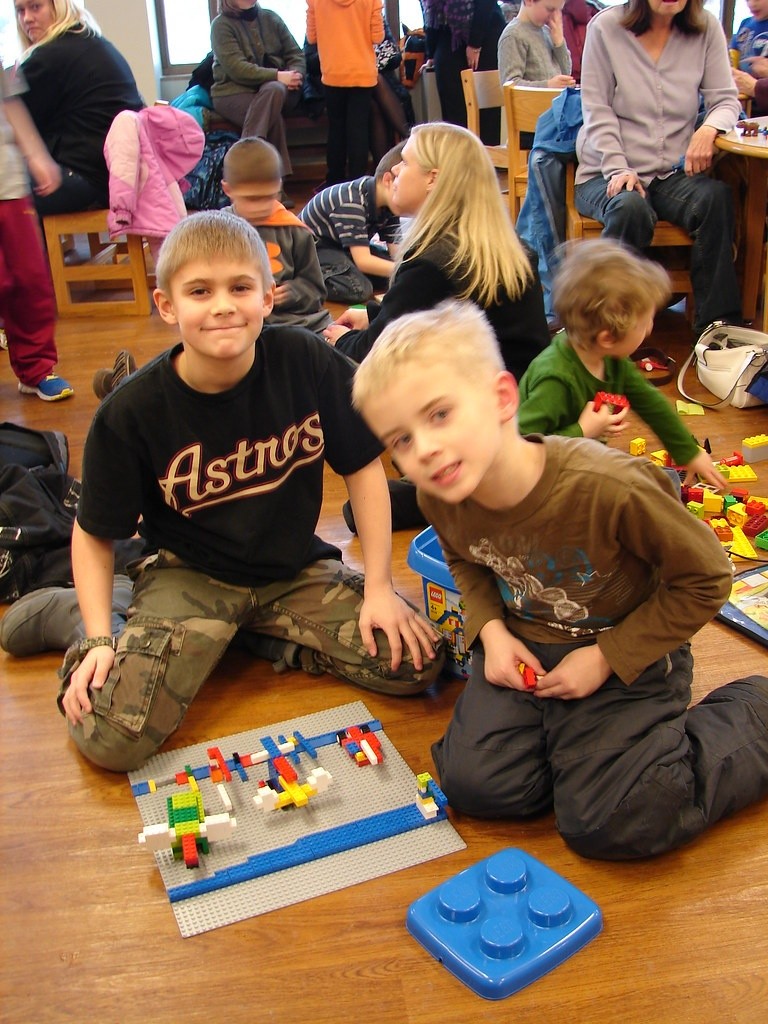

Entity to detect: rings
[471,59,474,64]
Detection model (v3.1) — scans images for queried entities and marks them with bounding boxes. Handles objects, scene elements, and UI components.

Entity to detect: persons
[0,49,76,403]
[1,210,453,774]
[301,1,386,187]
[573,0,743,367]
[418,0,507,173]
[304,14,410,167]
[348,296,767,867]
[214,138,408,337]
[497,0,576,150]
[322,123,552,535]
[517,237,729,492]
[726,0,768,106]
[14,0,144,219]
[209,1,306,211]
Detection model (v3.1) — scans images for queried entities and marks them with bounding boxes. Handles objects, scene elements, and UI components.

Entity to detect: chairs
[462,68,698,328]
[43,99,205,319]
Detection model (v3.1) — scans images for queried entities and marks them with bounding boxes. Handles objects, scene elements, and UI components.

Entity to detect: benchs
[203,107,381,182]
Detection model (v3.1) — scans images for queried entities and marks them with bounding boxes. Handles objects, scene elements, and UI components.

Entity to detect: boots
[0,574,134,657]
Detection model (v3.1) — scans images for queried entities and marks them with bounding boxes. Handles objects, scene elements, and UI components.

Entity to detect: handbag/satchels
[0,420,148,604]
[676,320,768,409]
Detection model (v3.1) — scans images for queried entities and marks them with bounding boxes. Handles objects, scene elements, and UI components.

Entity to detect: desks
[714,114,768,331]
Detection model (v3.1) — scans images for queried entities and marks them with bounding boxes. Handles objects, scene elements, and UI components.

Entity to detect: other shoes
[112,349,138,391]
[340,502,357,534]
[92,368,114,402]
[0,328,8,350]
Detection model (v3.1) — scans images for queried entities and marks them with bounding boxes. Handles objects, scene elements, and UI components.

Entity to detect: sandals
[628,344,672,386]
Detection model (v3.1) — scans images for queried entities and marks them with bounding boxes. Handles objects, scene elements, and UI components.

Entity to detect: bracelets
[468,47,481,53]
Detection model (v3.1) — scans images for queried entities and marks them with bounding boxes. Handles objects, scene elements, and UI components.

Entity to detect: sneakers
[18,371,73,401]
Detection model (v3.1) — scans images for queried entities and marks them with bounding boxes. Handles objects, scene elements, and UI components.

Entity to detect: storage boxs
[408,522,474,678]
